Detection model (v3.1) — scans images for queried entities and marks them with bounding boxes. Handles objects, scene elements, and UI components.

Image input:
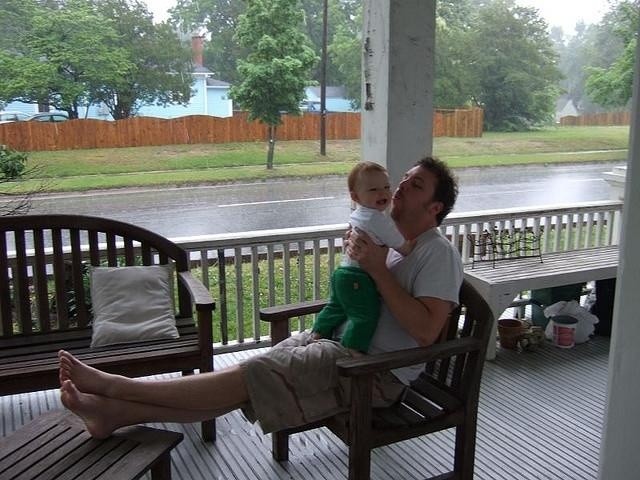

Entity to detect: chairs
[260,277,493,479]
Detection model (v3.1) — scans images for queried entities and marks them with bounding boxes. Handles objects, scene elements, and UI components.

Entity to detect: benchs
[1,215,216,443]
[467,245,621,360]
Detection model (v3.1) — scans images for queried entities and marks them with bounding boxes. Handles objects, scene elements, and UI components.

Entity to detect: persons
[57,156,464,442]
[310,160,416,349]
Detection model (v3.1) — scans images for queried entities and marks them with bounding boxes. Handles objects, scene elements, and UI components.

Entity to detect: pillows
[89,263,179,346]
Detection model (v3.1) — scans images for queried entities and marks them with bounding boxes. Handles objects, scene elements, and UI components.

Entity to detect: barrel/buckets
[550,315,578,349]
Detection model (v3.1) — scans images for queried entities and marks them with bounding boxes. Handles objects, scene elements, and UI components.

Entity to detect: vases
[497,318,522,348]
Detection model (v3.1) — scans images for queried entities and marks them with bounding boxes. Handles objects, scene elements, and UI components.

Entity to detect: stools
[0,408,183,479]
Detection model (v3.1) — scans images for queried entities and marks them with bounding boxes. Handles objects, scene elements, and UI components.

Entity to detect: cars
[0,110,70,124]
